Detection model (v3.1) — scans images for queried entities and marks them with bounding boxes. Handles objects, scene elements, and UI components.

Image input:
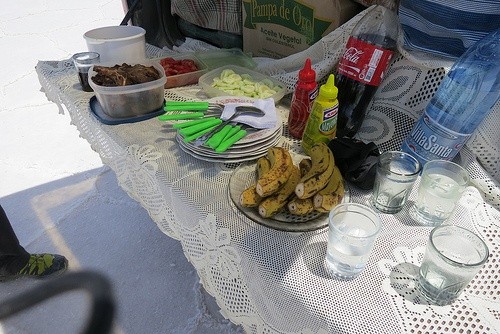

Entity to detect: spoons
[193,106,265,146]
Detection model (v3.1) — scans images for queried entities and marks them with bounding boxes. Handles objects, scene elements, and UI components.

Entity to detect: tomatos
[159,57,201,88]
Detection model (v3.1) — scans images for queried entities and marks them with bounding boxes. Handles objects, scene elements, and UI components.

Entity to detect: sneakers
[0,253,69,284]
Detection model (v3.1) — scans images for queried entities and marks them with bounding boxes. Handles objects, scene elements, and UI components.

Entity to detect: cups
[415,224,489,306]
[73,52,101,92]
[413,160,470,224]
[372,151,419,214]
[323,203,382,280]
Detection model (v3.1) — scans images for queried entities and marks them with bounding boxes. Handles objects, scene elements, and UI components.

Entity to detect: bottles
[332,0,400,139]
[301,74,339,155]
[400,27,500,177]
[288,58,318,140]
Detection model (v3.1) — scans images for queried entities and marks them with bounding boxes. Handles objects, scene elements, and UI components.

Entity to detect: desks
[32,34,500,334]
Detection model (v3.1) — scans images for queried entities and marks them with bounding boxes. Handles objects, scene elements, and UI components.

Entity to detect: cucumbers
[210,69,282,99]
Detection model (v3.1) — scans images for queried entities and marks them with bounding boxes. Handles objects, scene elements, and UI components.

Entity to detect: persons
[0,204,69,282]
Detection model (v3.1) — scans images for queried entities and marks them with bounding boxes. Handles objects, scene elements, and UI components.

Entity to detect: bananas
[239,143,345,218]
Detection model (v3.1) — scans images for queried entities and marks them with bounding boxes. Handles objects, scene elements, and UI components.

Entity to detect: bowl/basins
[151,51,209,89]
[198,65,287,106]
[87,59,167,118]
[84,26,147,63]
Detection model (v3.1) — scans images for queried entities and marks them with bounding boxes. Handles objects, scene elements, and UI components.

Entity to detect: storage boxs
[241,0,359,60]
[86,50,286,118]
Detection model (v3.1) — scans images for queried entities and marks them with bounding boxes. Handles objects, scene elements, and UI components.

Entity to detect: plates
[176,96,283,163]
[229,159,350,231]
